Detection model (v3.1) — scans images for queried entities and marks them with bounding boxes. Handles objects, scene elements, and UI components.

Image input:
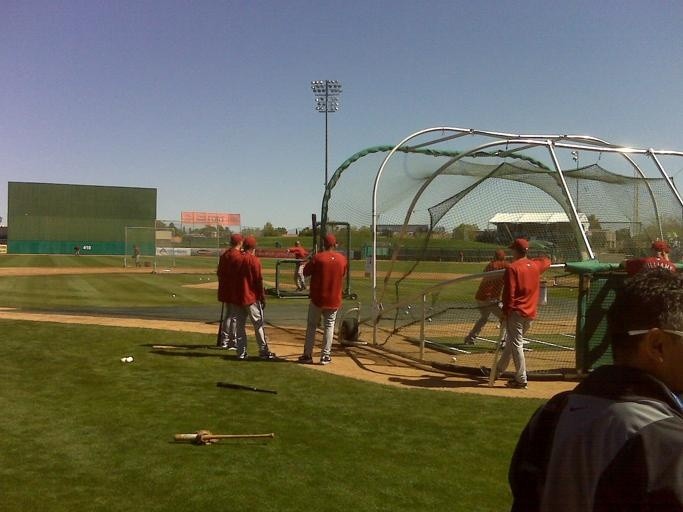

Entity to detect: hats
[243,238,256,248]
[231,235,242,243]
[325,234,336,245]
[651,240,671,253]
[508,238,529,251]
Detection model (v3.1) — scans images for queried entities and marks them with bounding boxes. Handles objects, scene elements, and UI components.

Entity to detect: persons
[621,241,677,278]
[464,248,512,348]
[133,245,141,268]
[74,246,81,256]
[232,237,275,359]
[298,233,348,366]
[285,241,310,292]
[216,234,244,349]
[507,266,683,512]
[481,238,551,388]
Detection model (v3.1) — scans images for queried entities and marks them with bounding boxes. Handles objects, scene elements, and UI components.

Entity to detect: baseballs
[408,306,411,309]
[405,311,409,315]
[121,356,134,363]
[497,325,499,328]
[428,318,432,322]
[452,357,457,362]
[172,295,176,297]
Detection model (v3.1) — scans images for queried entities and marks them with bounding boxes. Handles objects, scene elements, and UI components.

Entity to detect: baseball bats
[152,345,189,350]
[217,381,277,394]
[174,430,275,441]
[488,325,504,387]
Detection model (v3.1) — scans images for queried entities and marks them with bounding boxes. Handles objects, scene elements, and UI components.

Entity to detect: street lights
[570,150,579,213]
[308,74,344,194]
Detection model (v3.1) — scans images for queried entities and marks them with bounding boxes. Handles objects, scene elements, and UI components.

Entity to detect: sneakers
[481,365,497,381]
[507,380,527,386]
